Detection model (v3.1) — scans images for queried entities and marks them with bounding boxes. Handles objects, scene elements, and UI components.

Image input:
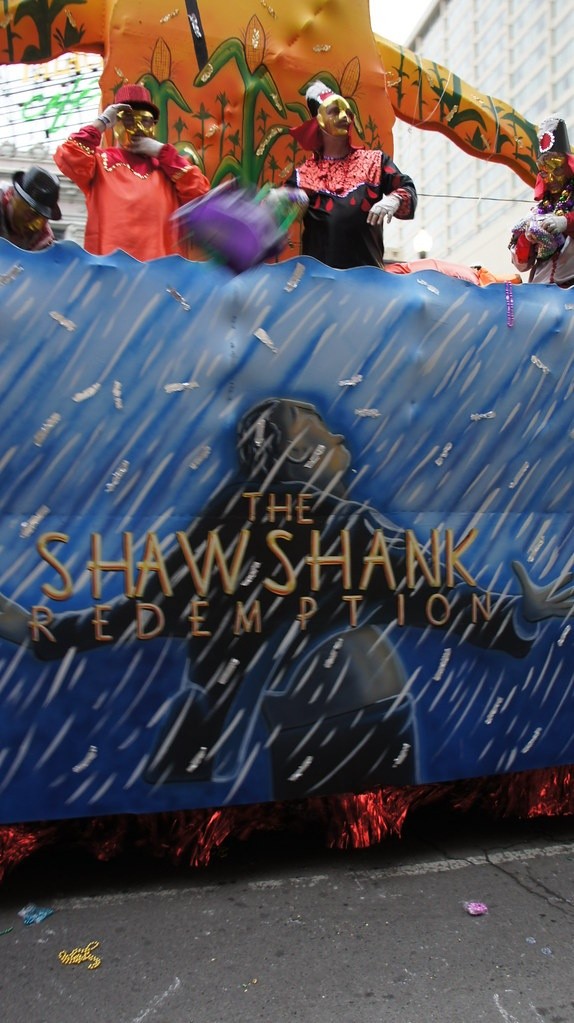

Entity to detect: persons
[53,85,211,263]
[283,79,417,269]
[507,118,574,289]
[0,163,63,252]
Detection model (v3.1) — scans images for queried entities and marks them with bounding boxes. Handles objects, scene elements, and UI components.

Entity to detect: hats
[114,84,160,125]
[288,81,365,150]
[12,165,62,218]
[534,116,574,201]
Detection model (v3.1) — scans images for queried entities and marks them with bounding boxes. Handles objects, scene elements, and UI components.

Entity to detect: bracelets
[98,116,110,131]
